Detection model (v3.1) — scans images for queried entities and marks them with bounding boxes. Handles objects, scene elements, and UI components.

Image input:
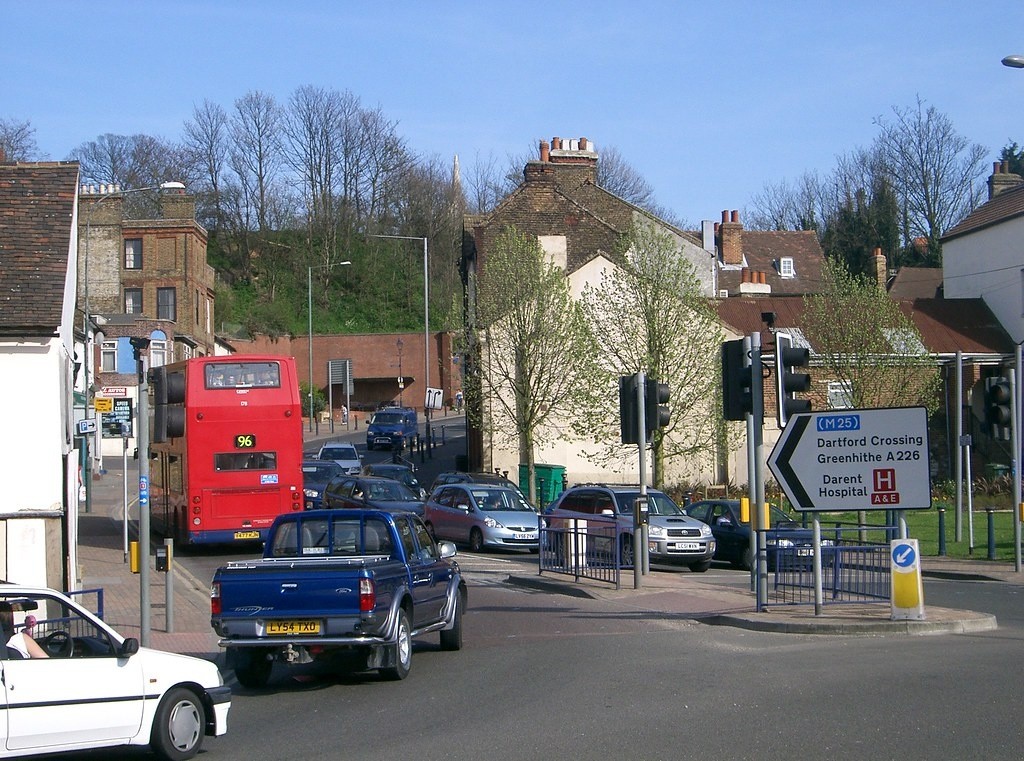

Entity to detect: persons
[456,389,463,406]
[357,487,372,498]
[341,404,347,425]
[0,601,49,658]
[716,512,731,525]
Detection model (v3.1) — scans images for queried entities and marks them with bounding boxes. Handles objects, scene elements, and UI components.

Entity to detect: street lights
[308,261,352,433]
[370,234,431,454]
[85,181,186,445]
[396,337,404,408]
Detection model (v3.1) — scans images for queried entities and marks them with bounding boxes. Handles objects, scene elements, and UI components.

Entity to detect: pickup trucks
[210,507,468,689]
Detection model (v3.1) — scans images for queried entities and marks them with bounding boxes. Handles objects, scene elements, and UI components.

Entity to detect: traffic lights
[992,383,1009,428]
[647,378,671,430]
[774,332,811,427]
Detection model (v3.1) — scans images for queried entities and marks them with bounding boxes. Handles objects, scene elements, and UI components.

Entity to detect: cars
[0,584,232,761]
[681,498,835,571]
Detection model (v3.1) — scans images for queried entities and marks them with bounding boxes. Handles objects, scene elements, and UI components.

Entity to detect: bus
[133,354,306,553]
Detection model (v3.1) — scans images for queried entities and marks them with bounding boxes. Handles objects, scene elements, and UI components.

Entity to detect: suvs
[365,409,421,450]
[544,483,717,572]
[423,470,550,555]
[302,441,426,521]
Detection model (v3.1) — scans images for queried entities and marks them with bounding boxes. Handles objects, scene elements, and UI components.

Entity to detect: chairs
[4,630,23,659]
[355,526,380,552]
[712,516,721,526]
[284,527,313,553]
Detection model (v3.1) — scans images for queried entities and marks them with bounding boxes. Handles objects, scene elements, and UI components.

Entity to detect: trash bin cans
[549,518,587,567]
[517,463,566,504]
